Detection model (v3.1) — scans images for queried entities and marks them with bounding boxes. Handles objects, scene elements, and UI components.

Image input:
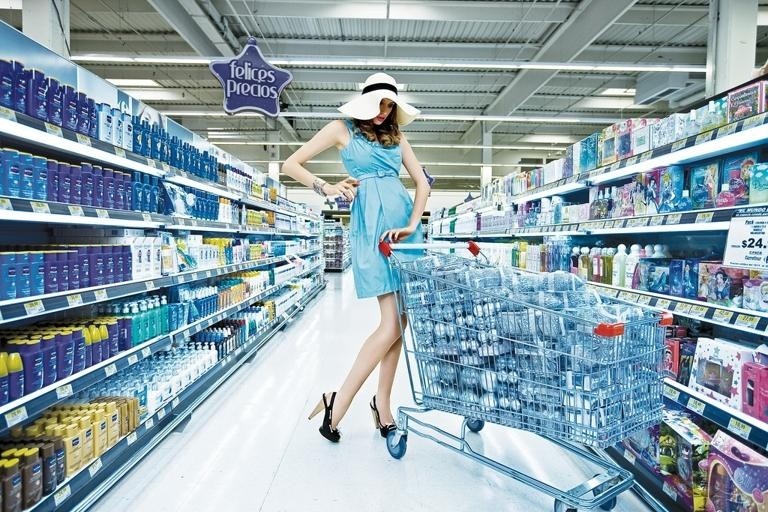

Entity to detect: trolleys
[376,240,674,511]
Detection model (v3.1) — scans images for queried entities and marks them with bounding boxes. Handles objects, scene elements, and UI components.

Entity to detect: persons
[280,72,430,445]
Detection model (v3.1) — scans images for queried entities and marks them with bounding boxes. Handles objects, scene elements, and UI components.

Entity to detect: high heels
[371,395,398,437]
[309,391,340,442]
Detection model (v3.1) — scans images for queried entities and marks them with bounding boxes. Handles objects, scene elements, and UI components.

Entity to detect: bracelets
[311,175,328,196]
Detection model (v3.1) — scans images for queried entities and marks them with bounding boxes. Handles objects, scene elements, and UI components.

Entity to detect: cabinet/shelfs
[0,101,351,512]
[430,118,766,510]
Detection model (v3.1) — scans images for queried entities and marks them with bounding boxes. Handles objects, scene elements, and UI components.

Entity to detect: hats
[336,73,421,126]
